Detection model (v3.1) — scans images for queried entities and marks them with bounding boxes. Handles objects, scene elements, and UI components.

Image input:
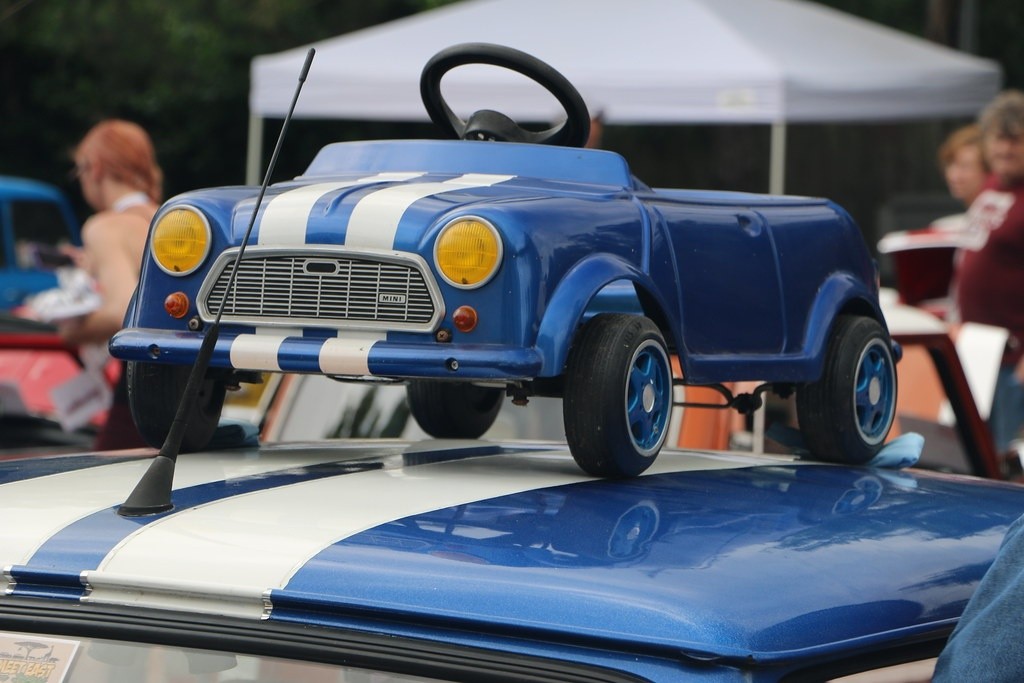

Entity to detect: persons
[57,120,165,409]
[937,89,1023,458]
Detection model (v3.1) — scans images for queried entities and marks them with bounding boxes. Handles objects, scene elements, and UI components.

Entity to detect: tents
[247,0,1002,194]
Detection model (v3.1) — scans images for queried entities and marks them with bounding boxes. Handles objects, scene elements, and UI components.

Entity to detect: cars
[107,41,899,483]
[0,435,1024,683]
[0,179,81,310]
